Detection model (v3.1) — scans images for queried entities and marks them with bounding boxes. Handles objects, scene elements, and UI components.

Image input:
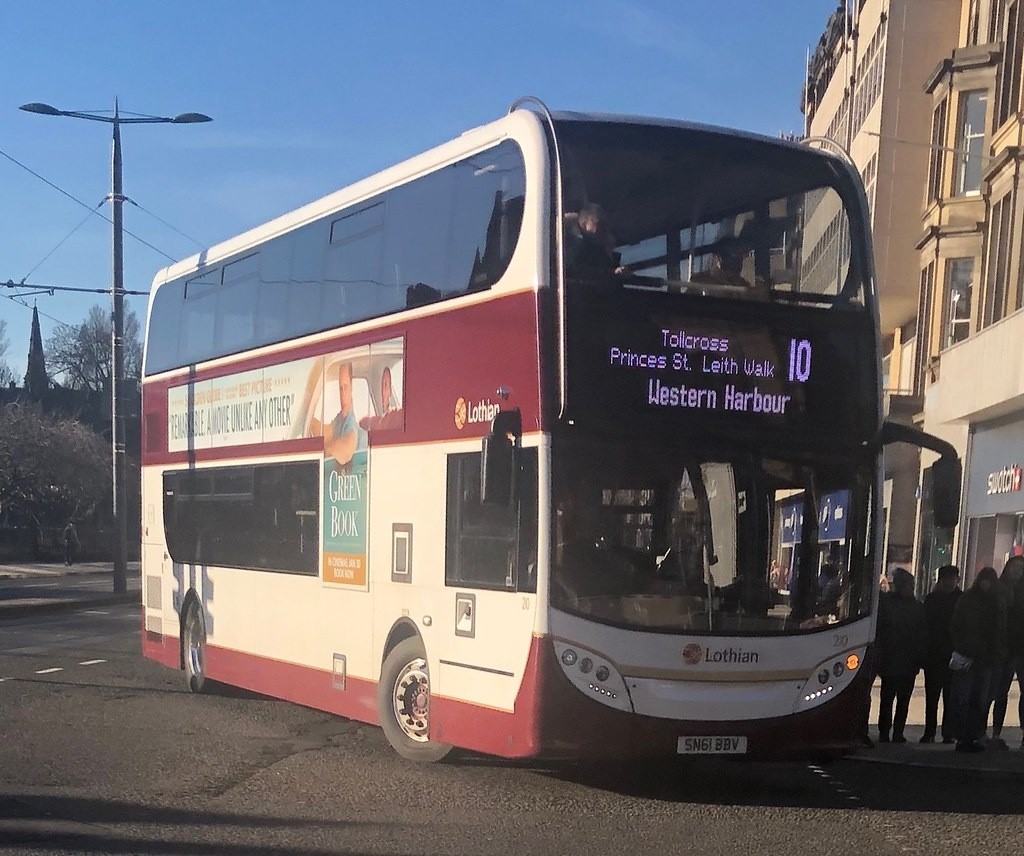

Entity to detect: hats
[892,568,914,587]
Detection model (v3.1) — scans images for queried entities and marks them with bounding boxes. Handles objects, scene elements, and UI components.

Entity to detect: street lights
[17,102,213,594]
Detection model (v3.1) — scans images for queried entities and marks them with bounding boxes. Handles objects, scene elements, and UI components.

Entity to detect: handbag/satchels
[948,651,974,672]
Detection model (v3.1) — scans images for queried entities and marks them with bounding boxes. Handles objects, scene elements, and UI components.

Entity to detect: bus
[140,97,959,767]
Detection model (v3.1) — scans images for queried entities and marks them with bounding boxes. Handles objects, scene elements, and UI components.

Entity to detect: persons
[323,362,358,465]
[565,202,630,291]
[812,553,1024,752]
[685,236,749,298]
[382,367,392,418]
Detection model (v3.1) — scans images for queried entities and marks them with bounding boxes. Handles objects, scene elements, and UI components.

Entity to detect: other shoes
[919,733,936,744]
[877,732,890,743]
[940,736,955,744]
[991,736,1009,749]
[891,732,908,742]
[973,737,998,748]
